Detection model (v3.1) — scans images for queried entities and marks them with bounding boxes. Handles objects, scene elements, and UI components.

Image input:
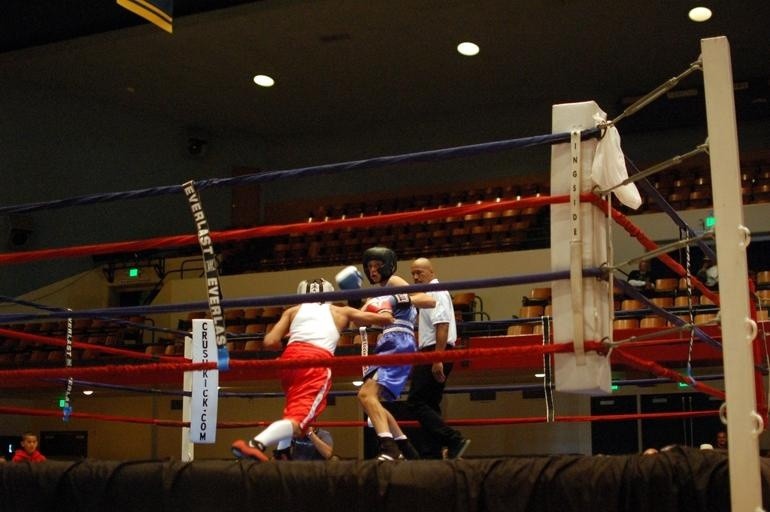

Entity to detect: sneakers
[443,434,470,460]
[272,446,293,461]
[230,438,269,462]
[369,436,422,461]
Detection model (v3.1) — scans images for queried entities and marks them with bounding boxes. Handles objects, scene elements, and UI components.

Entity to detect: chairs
[0,148,770,364]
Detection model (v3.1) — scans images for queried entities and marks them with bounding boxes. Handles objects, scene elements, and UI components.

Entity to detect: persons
[230,276,396,464]
[696,255,719,291]
[291,425,335,461]
[333,246,437,462]
[12,432,48,463]
[628,260,657,283]
[715,432,727,449]
[406,256,472,462]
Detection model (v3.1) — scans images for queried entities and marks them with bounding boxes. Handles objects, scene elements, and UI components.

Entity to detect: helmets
[296,278,335,304]
[362,246,398,283]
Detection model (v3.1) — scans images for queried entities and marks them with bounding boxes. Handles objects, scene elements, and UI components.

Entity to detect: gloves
[367,300,395,317]
[389,293,412,310]
[334,265,364,306]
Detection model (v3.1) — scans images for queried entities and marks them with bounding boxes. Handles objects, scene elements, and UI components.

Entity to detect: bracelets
[305,431,312,438]
